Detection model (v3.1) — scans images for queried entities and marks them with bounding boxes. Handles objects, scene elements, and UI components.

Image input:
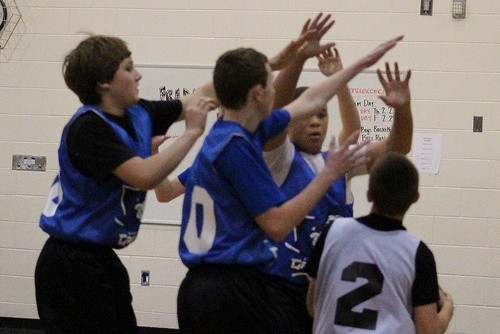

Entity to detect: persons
[149,136,195,203]
[262,11,413,334]
[35,30,317,334]
[177,35,405,334]
[306,152,455,333]
